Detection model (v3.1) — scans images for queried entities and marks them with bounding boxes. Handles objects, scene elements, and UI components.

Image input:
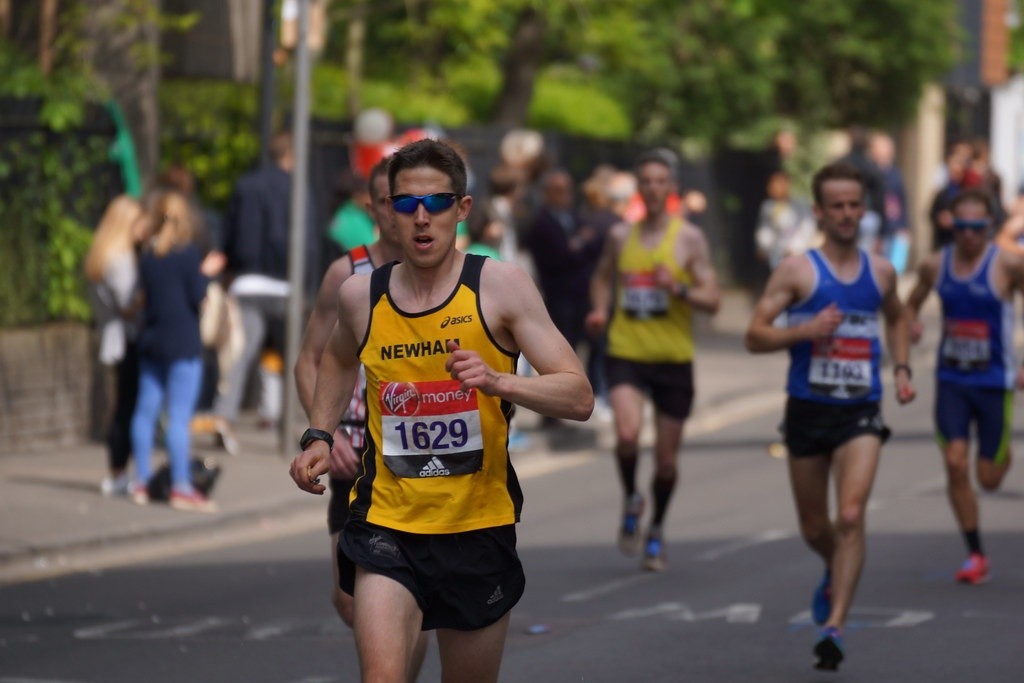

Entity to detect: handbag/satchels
[98,319,126,365]
[196,279,245,349]
[148,453,223,503]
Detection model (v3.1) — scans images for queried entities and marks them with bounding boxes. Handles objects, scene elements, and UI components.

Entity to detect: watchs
[300,427,334,453]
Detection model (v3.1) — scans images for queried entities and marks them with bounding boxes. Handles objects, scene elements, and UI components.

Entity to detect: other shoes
[102,474,130,496]
[128,480,150,506]
[167,487,220,512]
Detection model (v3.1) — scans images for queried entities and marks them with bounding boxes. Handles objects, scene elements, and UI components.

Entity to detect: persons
[737,131,912,329]
[212,123,380,454]
[906,191,1024,584]
[468,149,706,452]
[745,165,916,672]
[586,150,719,573]
[928,138,1024,293]
[84,169,244,512]
[288,137,594,683]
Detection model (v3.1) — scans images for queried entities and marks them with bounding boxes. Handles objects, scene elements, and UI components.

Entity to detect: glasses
[390,192,466,214]
[951,218,993,232]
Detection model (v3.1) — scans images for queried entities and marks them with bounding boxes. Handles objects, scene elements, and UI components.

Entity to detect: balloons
[352,109,439,180]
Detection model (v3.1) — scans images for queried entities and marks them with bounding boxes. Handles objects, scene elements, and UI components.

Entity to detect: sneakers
[955,552,993,584]
[809,570,833,624]
[810,626,846,671]
[615,490,647,547]
[640,527,666,573]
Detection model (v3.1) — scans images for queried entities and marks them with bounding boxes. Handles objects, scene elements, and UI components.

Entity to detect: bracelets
[894,364,911,380]
